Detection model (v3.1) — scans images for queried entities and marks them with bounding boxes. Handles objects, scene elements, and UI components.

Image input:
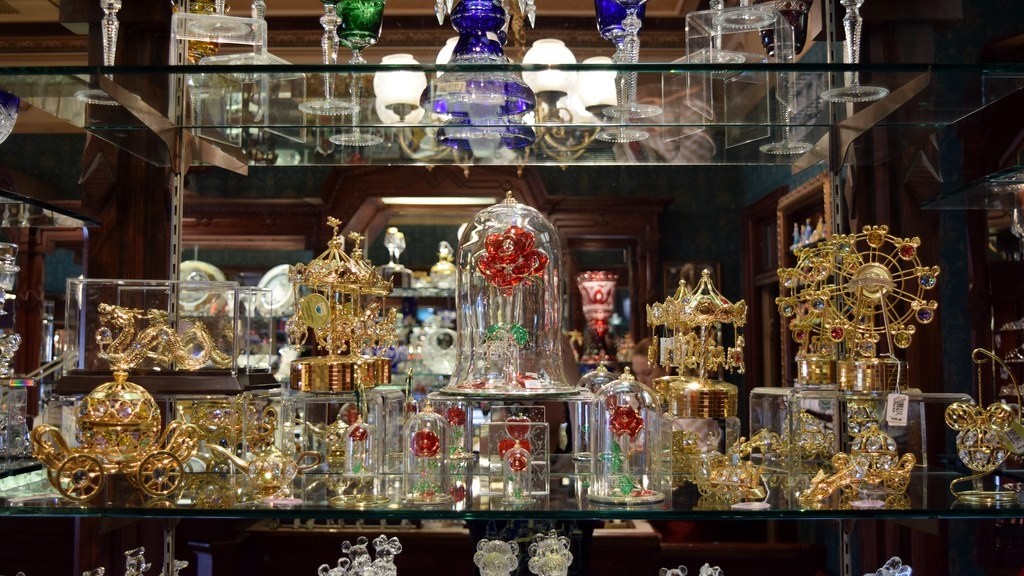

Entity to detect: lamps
[370,29,623,175]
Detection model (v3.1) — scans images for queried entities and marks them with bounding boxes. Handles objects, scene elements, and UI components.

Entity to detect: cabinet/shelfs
[0,0,1024,576]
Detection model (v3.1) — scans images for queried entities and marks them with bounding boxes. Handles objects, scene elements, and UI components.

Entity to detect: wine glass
[685,0,777,74]
[298,0,385,149]
[820,0,888,103]
[592,0,663,144]
[754,0,815,156]
